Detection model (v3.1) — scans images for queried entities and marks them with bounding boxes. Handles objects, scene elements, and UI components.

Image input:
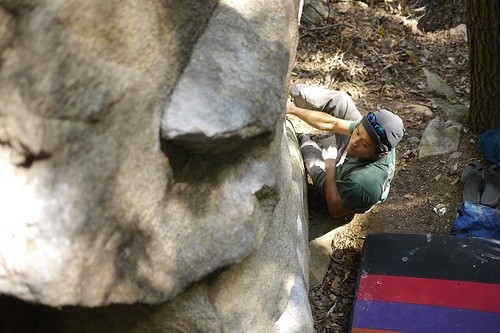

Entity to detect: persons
[287,85,405,219]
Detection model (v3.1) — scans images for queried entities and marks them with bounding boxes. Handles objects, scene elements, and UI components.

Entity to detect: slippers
[482,164,500,207]
[460,158,482,203]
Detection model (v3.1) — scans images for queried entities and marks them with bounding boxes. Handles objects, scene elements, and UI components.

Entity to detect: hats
[361,110,405,154]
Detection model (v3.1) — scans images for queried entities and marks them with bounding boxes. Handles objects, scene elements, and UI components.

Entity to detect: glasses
[367,112,392,152]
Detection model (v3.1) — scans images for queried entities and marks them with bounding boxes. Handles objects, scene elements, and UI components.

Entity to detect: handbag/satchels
[451,201,500,242]
[481,129,500,164]
